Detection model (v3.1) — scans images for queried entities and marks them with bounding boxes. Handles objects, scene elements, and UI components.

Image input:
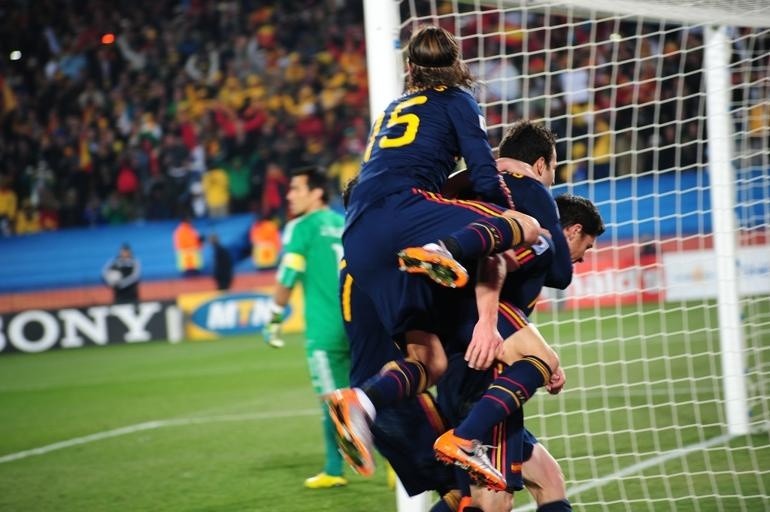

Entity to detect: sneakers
[432,427,510,494]
[319,386,378,478]
[301,470,350,489]
[395,239,472,291]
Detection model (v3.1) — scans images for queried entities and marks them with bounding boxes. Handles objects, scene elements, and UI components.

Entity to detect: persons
[0,0,371,242]
[208,235,234,293]
[263,165,351,489]
[101,243,141,303]
[322,24,602,511]
[249,210,285,273]
[172,214,206,276]
[398,2,770,186]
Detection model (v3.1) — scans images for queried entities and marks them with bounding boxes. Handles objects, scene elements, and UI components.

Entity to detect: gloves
[260,303,290,349]
